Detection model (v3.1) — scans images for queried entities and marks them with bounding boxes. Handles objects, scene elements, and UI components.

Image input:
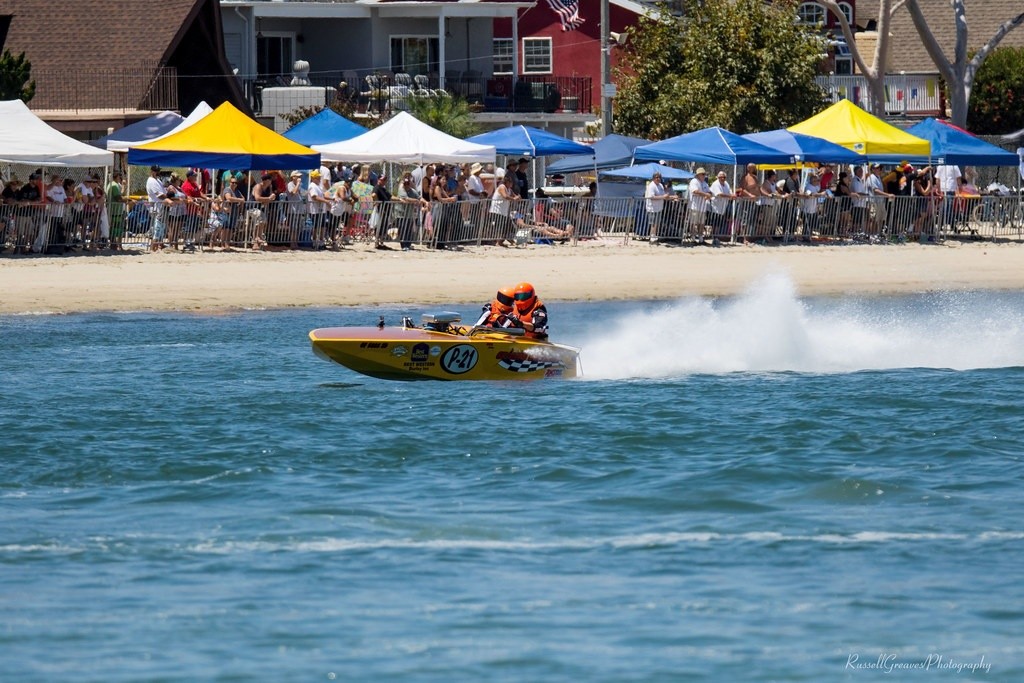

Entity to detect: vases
[561,96,578,110]
[369,99,386,114]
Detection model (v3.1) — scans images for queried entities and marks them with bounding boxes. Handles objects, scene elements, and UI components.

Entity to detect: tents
[866,117,1021,195]
[128,101,321,249]
[461,125,601,221]
[630,126,797,245]
[105,101,215,243]
[309,110,498,195]
[545,134,658,184]
[740,130,869,193]
[280,108,371,190]
[88,110,187,243]
[0,99,115,207]
[756,99,936,235]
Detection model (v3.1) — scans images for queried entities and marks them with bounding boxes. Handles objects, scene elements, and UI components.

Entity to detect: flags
[547,0,585,32]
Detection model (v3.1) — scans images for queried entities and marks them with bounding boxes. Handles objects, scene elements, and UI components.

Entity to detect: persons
[478,282,549,341]
[644,164,979,246]
[146,158,598,253]
[0,168,139,254]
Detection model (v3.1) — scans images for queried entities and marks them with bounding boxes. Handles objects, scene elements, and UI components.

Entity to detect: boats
[308,300,583,381]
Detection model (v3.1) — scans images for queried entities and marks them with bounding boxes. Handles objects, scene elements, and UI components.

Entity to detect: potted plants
[561,71,594,96]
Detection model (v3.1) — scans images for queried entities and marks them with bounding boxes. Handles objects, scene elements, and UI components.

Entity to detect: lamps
[446,18,453,37]
[256,17,264,38]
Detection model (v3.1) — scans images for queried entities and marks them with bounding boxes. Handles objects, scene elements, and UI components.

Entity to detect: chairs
[366,73,448,111]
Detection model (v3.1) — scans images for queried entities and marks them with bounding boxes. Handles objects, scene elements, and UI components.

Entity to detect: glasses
[231,182,238,184]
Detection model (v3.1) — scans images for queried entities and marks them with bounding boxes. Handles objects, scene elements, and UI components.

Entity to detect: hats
[152,165,162,172]
[290,171,302,177]
[345,177,353,182]
[518,158,530,165]
[870,163,884,170]
[471,163,483,175]
[895,160,909,172]
[36,169,49,177]
[694,168,710,178]
[4,176,24,187]
[29,173,39,180]
[85,176,94,181]
[186,171,196,177]
[310,171,322,179]
[904,164,917,171]
[506,159,519,169]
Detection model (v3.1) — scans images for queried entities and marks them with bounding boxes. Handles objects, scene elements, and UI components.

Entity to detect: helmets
[496,288,514,312]
[514,282,535,310]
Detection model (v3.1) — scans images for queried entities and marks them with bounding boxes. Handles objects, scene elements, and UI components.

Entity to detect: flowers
[368,90,387,99]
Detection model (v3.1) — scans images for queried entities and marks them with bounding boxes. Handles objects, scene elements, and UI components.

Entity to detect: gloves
[506,313,523,327]
